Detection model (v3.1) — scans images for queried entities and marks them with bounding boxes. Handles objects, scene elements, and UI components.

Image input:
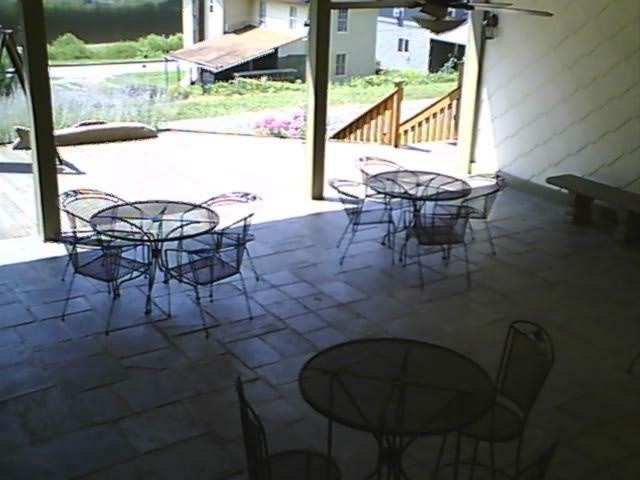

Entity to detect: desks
[298,334,497,480]
[90,202,219,317]
[364,170,473,264]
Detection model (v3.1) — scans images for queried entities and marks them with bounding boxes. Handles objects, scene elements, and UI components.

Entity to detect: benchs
[544,172,640,251]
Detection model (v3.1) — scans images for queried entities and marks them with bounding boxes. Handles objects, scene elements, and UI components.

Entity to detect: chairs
[177,191,265,301]
[509,438,560,480]
[230,376,345,480]
[432,318,559,480]
[54,186,148,284]
[56,229,160,334]
[354,157,421,243]
[394,200,474,297]
[165,229,255,338]
[433,172,506,258]
[328,175,406,264]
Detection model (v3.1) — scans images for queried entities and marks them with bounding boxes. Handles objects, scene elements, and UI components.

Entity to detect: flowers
[246,102,311,141]
